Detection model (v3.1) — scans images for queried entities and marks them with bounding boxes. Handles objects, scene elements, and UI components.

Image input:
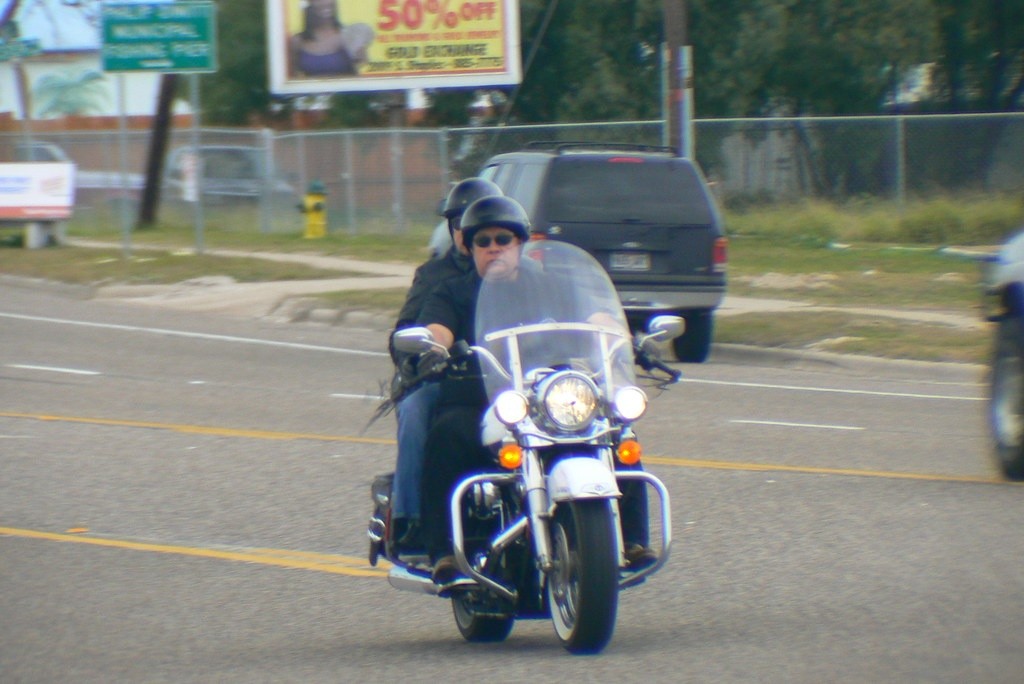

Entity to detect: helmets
[460,194,530,250]
[443,176,506,217]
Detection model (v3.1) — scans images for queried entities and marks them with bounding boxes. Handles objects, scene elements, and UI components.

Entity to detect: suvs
[426,139,732,363]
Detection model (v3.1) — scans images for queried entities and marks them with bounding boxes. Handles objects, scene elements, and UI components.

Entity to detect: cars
[161,142,292,209]
[974,230,1023,485]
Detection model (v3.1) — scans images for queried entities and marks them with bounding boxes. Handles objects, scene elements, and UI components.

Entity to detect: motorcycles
[363,239,687,656]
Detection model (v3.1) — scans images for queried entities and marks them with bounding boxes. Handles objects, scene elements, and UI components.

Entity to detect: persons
[388,177,658,584]
[289,0,373,79]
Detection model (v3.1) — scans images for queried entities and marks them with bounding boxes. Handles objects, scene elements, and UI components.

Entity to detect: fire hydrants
[293,180,333,240]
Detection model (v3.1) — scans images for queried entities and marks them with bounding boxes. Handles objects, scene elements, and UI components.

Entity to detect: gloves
[417,349,449,383]
[633,332,661,372]
[400,351,421,390]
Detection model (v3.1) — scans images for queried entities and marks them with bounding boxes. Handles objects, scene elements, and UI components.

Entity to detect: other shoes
[625,545,658,572]
[434,557,465,596]
[396,539,430,563]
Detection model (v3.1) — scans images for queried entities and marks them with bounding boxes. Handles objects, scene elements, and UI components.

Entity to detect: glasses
[472,233,516,248]
